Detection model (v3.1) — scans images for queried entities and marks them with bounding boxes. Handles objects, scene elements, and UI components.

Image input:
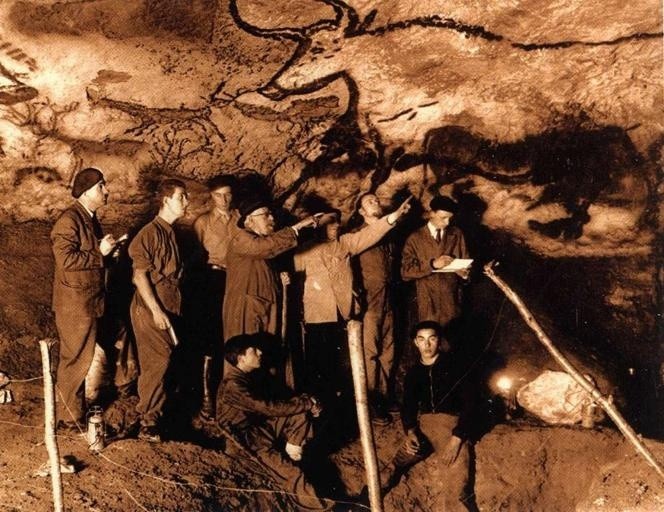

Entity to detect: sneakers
[58,420,87,434]
[370,403,401,426]
[138,426,161,443]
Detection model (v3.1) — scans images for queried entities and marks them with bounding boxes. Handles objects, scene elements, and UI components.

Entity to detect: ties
[436,228,441,244]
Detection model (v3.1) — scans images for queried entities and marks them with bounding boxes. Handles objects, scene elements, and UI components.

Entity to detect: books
[430,256,474,274]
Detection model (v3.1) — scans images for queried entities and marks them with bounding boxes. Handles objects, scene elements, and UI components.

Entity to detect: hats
[430,196,456,214]
[237,200,264,229]
[72,168,103,198]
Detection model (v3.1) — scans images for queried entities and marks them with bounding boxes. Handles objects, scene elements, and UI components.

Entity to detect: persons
[126,178,192,442]
[275,192,414,404]
[191,172,241,421]
[398,195,472,368]
[345,193,396,427]
[359,319,478,511]
[212,332,340,511]
[221,197,325,378]
[48,166,122,434]
